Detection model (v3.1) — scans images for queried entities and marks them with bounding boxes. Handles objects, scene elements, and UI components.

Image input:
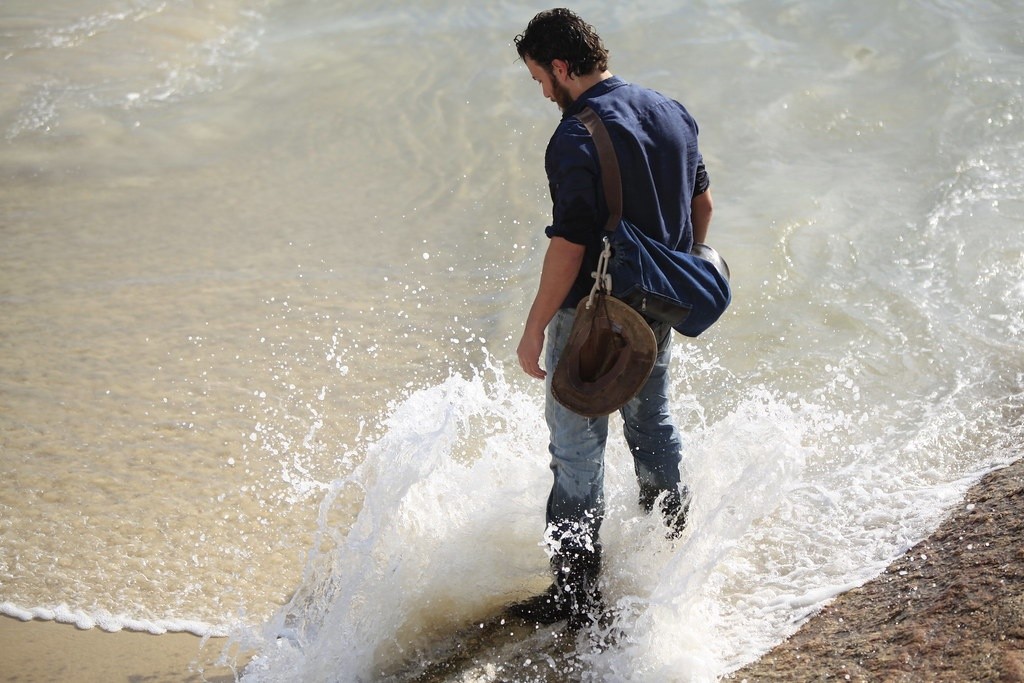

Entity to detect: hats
[550,294,658,420]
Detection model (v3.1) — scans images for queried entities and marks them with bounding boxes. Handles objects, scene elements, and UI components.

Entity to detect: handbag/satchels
[608,229,732,338]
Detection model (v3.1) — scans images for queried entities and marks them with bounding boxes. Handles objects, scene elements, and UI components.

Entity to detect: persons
[497,6,713,632]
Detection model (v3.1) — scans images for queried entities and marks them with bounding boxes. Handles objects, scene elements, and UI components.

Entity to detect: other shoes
[510,591,604,631]
